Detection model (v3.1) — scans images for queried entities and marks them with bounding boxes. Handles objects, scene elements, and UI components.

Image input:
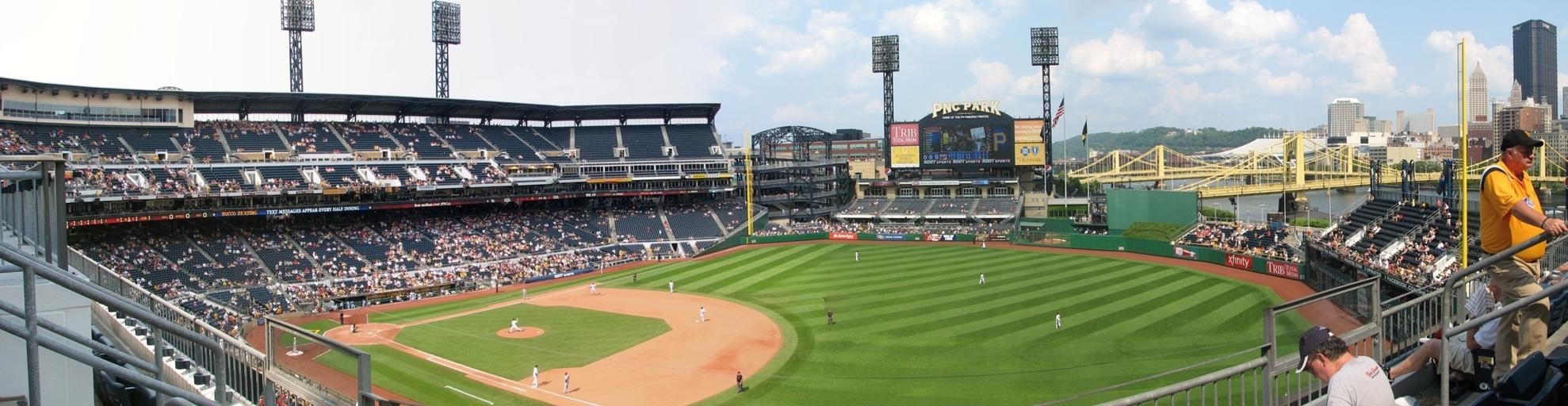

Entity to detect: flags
[1080,120,1087,146]
[1039,125,1045,144]
[1051,98,1065,128]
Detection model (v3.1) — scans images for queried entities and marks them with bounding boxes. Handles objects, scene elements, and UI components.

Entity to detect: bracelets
[1540,215,1550,230]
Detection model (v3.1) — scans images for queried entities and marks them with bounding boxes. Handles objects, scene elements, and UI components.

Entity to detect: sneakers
[1378,362,1395,386]
[1449,382,1467,400]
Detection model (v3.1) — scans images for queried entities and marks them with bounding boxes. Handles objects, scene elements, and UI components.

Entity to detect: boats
[1545,209,1565,215]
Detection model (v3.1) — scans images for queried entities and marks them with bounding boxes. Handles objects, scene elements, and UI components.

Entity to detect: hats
[1501,129,1544,154]
[1295,325,1335,374]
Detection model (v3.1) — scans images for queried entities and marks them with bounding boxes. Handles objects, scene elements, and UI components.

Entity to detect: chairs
[95,366,154,405]
[0,115,1024,331]
[1179,198,1568,406]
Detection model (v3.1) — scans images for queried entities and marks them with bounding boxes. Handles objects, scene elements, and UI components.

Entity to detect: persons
[589,282,597,294]
[854,251,860,262]
[1375,259,1568,400]
[1064,194,1108,236]
[1055,311,1061,328]
[531,364,539,388]
[736,371,743,393]
[532,189,741,277]
[741,152,1025,251]
[253,386,309,406]
[1293,325,1397,406]
[1172,179,1491,325]
[562,372,570,394]
[509,317,524,333]
[699,307,706,322]
[1,104,359,357]
[827,309,834,325]
[668,280,673,293]
[940,126,991,152]
[979,272,985,284]
[633,273,637,284]
[1477,128,1567,398]
[359,119,531,308]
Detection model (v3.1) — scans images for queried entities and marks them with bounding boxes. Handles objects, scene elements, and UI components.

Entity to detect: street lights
[872,35,900,168]
[1031,26,1058,195]
[429,1,461,124]
[280,0,315,121]
